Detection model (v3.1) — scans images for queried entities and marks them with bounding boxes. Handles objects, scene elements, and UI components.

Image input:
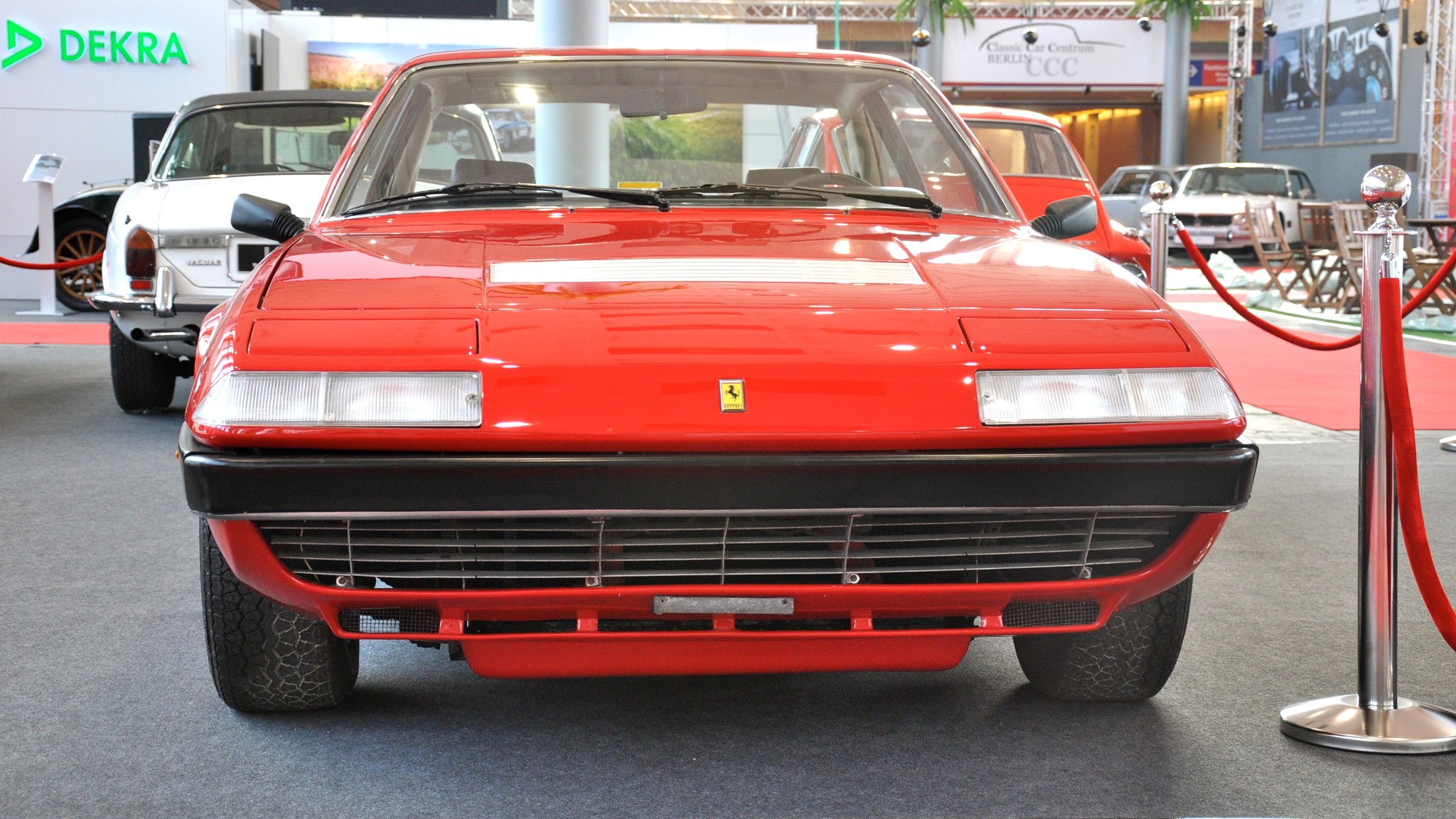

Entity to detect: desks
[1406,218,1456,294]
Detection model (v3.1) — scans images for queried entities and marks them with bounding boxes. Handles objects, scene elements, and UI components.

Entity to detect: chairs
[1246,198,1456,316]
[456,157,822,194]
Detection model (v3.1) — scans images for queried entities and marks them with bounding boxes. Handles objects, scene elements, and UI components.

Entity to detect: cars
[452,108,531,153]
[778,105,1152,283]
[175,45,1259,714]
[17,89,501,415]
[1098,163,1318,262]
